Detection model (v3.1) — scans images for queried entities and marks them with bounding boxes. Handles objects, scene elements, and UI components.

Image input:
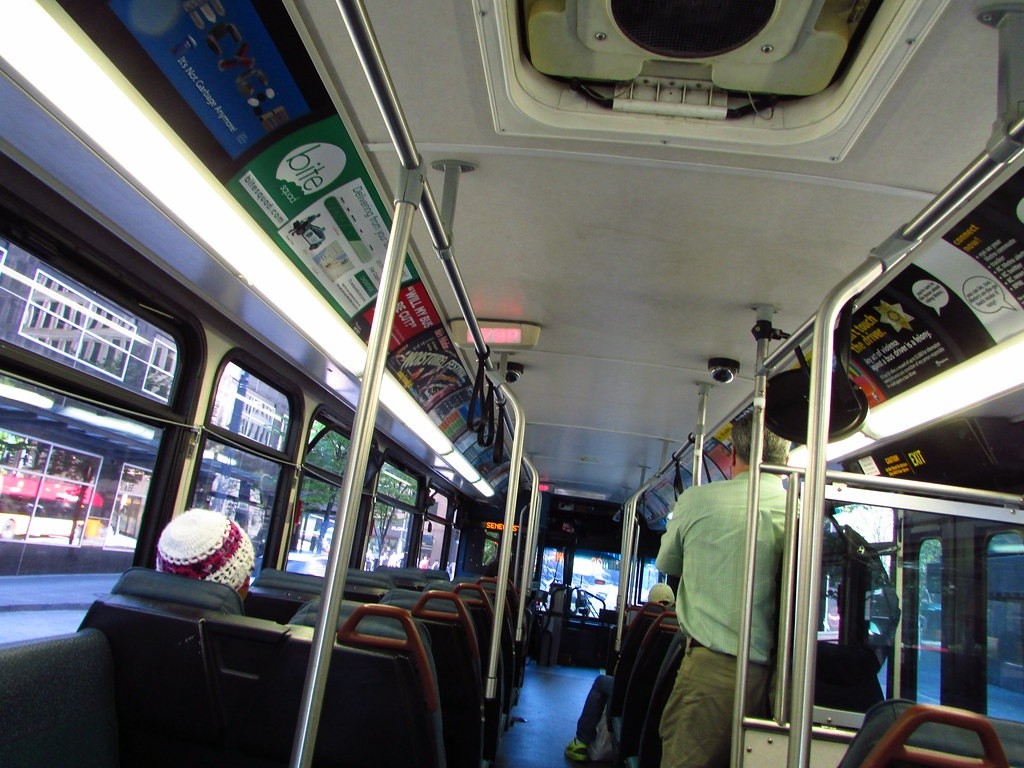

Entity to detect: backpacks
[776,504,901,707]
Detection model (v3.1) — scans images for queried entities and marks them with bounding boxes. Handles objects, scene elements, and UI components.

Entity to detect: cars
[247,554,331,587]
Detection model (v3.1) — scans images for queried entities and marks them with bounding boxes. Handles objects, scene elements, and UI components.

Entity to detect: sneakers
[565,738,588,761]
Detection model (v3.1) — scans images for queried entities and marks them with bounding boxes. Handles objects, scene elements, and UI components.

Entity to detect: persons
[156,509,257,603]
[565,583,674,760]
[419,555,452,577]
[366,549,408,572]
[486,549,497,566]
[655,413,802,768]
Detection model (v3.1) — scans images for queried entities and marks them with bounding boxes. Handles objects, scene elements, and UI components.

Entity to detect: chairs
[0,625,119,768]
[111,564,532,768]
[836,699,1024,768]
[610,609,679,758]
[636,630,685,768]
[605,601,674,733]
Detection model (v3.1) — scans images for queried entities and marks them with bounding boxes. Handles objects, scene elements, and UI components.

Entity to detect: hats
[648,582,676,608]
[156,509,255,591]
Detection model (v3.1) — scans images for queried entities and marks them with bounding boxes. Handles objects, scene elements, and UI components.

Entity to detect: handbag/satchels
[589,704,613,761]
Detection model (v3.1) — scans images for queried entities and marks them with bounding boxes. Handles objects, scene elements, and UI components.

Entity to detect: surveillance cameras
[498,362,524,382]
[708,358,740,385]
